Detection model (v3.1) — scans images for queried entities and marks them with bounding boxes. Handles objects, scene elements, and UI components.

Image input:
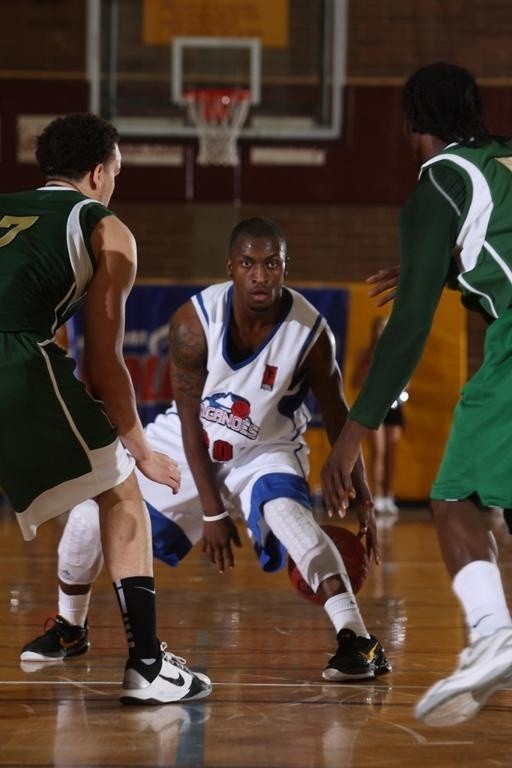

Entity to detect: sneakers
[16,616,94,666]
[411,629,511,729]
[120,658,216,708]
[320,630,394,684]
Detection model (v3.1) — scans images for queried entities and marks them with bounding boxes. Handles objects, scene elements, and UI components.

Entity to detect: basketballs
[288,526,368,605]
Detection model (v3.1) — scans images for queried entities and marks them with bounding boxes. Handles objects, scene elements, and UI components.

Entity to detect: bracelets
[200,510,229,523]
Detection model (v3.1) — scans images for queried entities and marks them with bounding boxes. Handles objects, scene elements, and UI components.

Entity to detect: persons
[350,314,402,517]
[0,109,218,711]
[114,700,211,768]
[302,53,511,731]
[28,659,395,768]
[17,215,398,685]
